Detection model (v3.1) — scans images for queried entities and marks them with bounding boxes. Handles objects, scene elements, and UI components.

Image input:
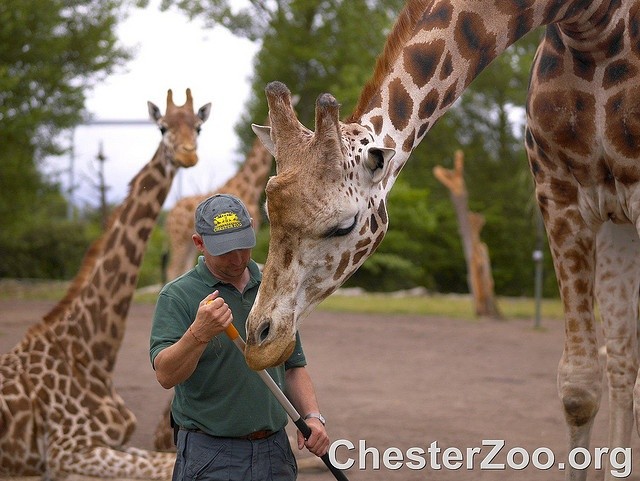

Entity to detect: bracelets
[189,326,210,343]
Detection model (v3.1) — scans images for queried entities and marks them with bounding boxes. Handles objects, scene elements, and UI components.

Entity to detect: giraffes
[162,94,301,284]
[244,0,640,466]
[1,89,327,480]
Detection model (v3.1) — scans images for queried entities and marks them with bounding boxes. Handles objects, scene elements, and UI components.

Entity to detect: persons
[150,193,331,480]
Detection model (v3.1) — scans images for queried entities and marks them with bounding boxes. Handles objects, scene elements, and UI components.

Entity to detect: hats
[195,195,256,256]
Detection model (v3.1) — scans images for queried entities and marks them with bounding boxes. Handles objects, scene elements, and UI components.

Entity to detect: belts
[239,430,274,440]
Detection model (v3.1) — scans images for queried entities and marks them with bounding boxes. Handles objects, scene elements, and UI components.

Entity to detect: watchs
[305,412,326,426]
